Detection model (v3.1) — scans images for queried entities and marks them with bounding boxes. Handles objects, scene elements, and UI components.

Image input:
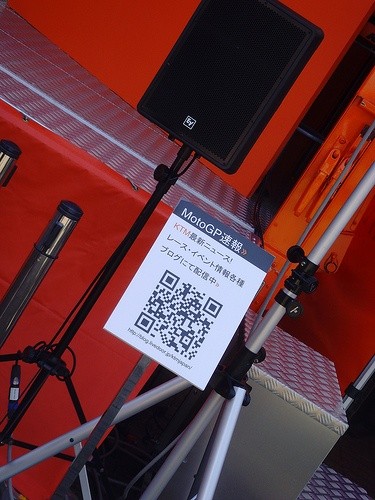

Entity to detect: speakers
[134,1,323,174]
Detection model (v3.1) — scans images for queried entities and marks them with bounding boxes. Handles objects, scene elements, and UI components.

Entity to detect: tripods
[1,109,375,500]
